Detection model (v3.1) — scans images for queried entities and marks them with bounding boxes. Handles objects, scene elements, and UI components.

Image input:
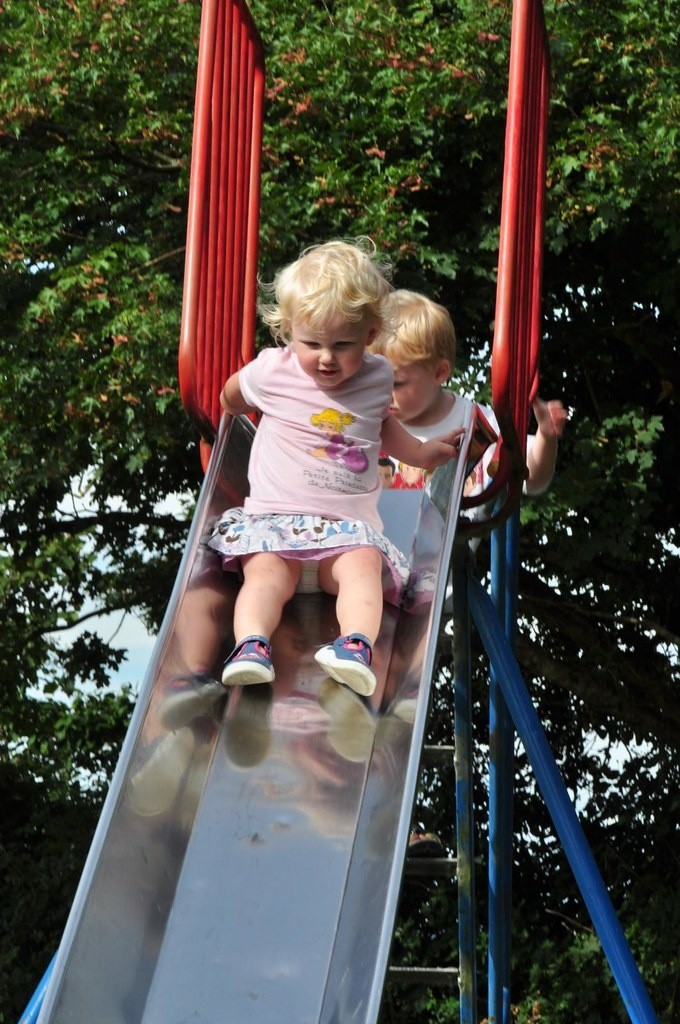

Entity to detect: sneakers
[317,634,376,692]
[223,639,276,685]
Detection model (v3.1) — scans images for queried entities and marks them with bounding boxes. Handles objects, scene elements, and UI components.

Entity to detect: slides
[23,400,477,1013]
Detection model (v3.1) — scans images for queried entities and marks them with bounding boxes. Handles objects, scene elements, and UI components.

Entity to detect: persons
[204,240,466,698]
[365,288,566,558]
[124,554,444,835]
